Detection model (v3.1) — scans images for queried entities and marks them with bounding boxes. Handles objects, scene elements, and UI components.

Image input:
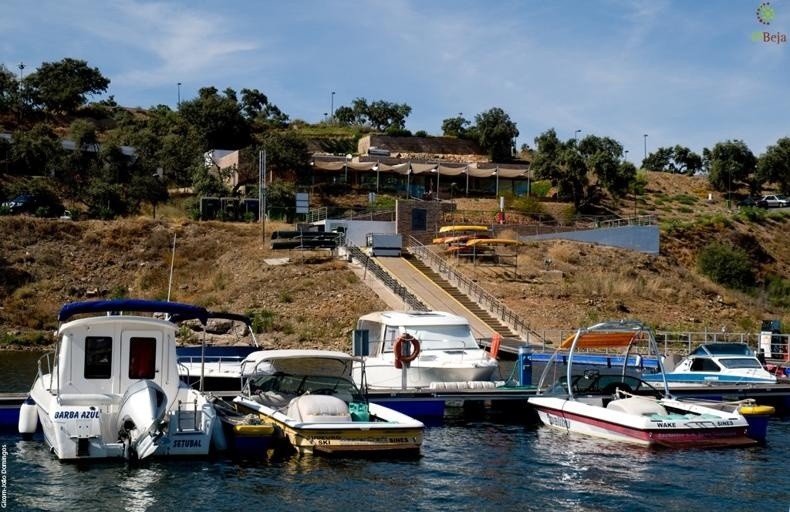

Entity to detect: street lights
[177,83,181,110]
[575,130,581,147]
[17,61,27,130]
[625,150,629,161]
[331,92,336,118]
[324,113,328,123]
[644,134,648,159]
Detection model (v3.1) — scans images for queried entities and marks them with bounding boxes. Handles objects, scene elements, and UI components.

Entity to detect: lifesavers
[394,334,420,362]
[739,405,776,414]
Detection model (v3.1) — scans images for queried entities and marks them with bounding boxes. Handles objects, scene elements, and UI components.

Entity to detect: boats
[19,232,790,469]
[439,225,488,233]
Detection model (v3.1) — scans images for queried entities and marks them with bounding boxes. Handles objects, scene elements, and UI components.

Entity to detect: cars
[736,196,768,211]
[2,194,59,215]
[761,194,790,208]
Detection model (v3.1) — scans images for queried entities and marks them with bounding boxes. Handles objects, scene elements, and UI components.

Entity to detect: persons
[757,348,767,367]
